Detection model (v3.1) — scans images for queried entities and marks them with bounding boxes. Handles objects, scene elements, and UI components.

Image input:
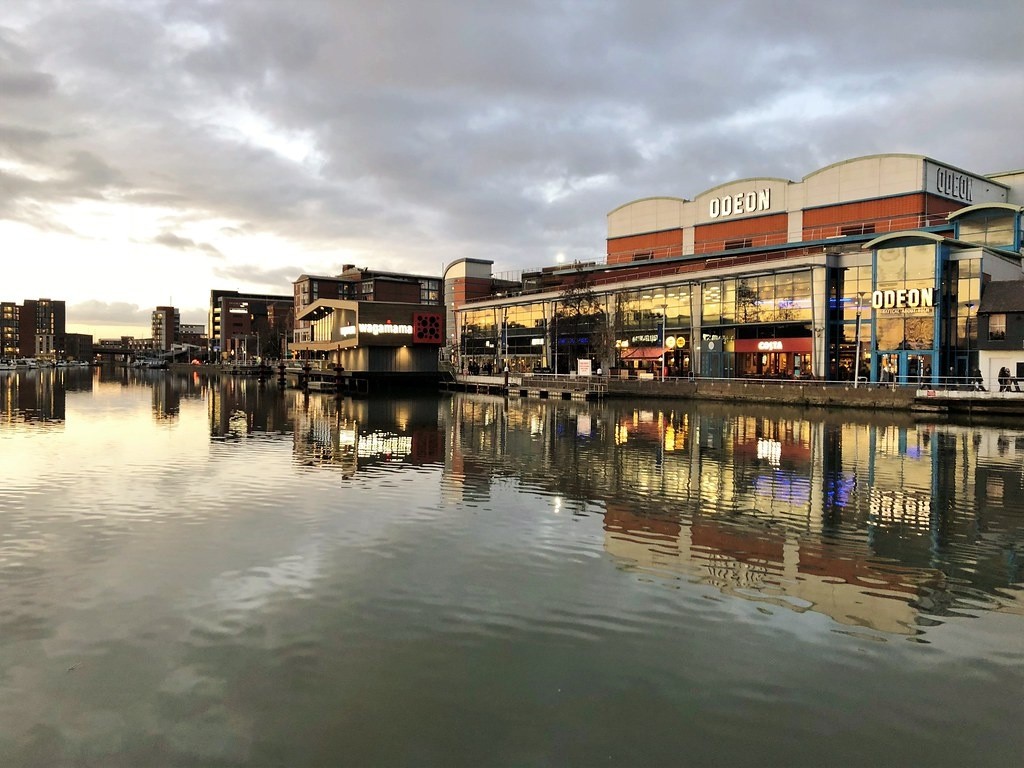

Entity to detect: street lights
[661,304,669,383]
[854,291,868,389]
[244,334,248,366]
[965,303,974,387]
[255,332,259,358]
[504,315,510,369]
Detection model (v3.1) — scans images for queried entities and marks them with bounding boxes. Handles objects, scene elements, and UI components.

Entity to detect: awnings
[618,346,672,360]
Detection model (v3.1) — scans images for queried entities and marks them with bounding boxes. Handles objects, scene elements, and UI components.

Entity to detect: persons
[219,354,345,372]
[596,366,603,383]
[765,366,811,380]
[535,365,554,373]
[837,357,1016,392]
[459,358,493,376]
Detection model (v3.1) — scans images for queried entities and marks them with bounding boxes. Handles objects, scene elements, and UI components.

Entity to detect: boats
[0,357,91,371]
[131,356,170,369]
